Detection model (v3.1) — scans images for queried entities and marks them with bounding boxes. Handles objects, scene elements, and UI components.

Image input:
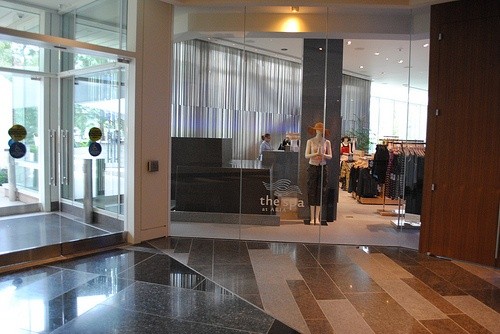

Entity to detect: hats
[307,122,330,137]
[341,136,351,141]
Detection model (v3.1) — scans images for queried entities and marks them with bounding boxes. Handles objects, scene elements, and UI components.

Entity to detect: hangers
[377,139,426,156]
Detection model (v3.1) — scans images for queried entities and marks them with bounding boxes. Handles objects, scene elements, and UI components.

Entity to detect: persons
[340,136,353,156]
[304,121,334,227]
[260,133,274,169]
